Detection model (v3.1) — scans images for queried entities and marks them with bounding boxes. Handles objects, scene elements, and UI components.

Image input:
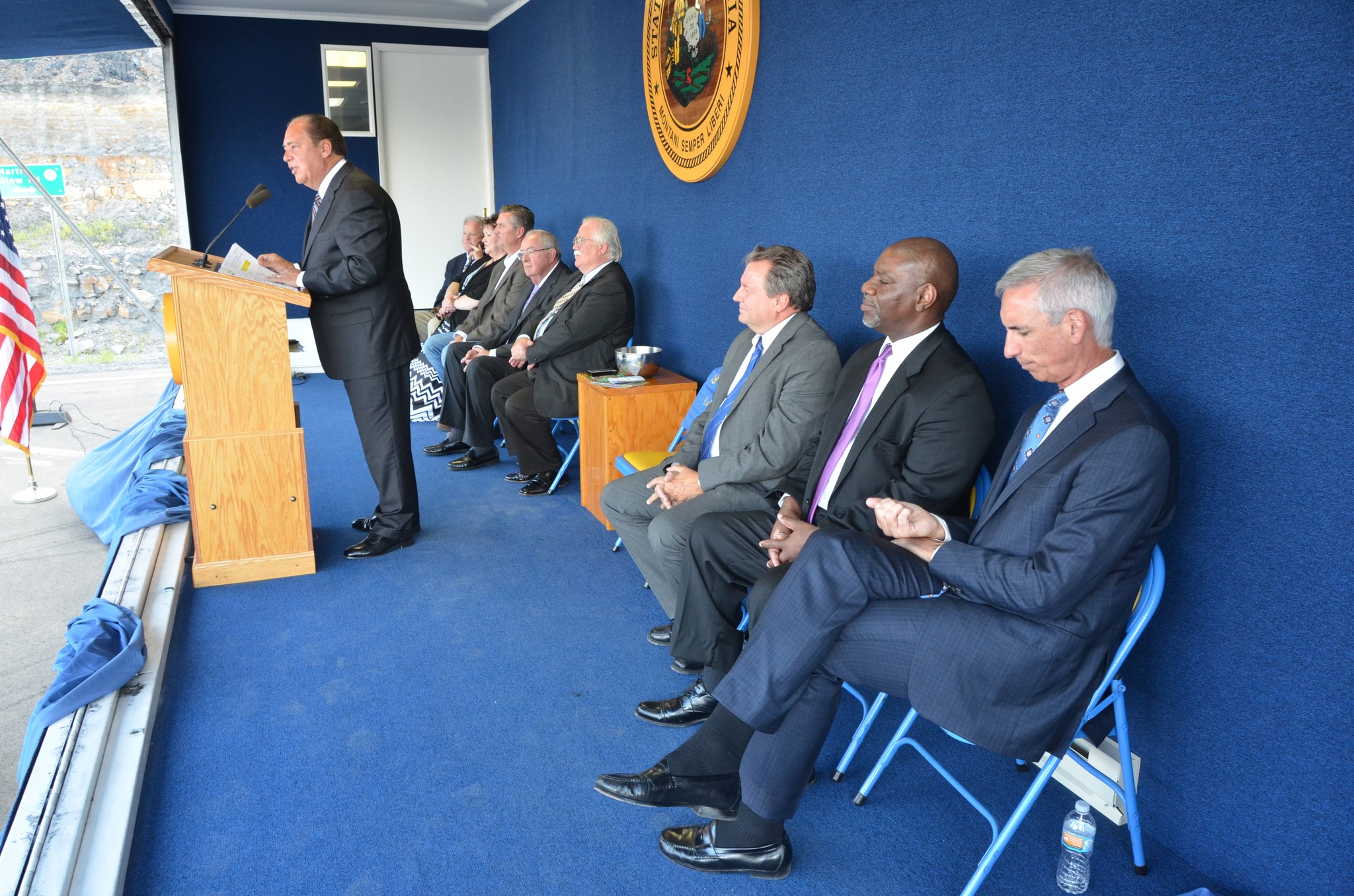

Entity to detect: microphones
[194,183,266,265]
[193,189,271,268]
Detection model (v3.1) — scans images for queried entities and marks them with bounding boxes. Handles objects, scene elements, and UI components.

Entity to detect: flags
[0,189,47,456]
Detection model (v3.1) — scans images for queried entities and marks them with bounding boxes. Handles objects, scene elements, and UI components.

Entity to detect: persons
[424,214,508,437]
[601,245,890,675]
[414,214,485,341]
[634,237,995,727]
[593,245,1181,878]
[422,205,574,470]
[491,214,636,496]
[257,113,421,559]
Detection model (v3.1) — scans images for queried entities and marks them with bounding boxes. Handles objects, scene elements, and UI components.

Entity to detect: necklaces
[492,259,494,262]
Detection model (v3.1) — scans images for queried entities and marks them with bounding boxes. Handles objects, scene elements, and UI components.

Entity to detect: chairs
[850,544,1166,896]
[494,337,750,633]
[832,467,990,784]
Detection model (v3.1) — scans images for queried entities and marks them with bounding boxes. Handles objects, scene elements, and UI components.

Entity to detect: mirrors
[320,44,377,138]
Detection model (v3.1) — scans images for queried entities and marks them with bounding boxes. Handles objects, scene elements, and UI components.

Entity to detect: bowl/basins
[614,346,662,377]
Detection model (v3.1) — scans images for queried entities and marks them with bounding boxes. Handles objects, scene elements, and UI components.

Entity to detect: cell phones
[586,367,617,378]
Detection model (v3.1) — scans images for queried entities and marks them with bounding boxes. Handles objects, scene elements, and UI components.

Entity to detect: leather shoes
[351,514,380,532]
[447,445,500,470]
[343,533,415,559]
[647,624,673,646]
[593,756,742,822]
[504,472,539,483]
[671,656,706,675]
[657,819,792,880]
[634,677,718,729]
[518,470,569,496]
[421,437,471,456]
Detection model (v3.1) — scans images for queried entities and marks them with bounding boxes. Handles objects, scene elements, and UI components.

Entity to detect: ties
[807,346,893,525]
[312,193,322,227]
[697,336,763,466]
[1005,389,1069,486]
[533,276,586,344]
[505,285,539,345]
[462,259,475,272]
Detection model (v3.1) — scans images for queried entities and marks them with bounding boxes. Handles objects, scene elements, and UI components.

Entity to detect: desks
[576,368,698,532]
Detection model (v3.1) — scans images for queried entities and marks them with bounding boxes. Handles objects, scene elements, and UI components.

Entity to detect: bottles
[1057,800,1097,895]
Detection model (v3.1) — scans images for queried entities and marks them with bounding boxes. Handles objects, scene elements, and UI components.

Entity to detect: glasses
[572,237,595,248]
[517,248,550,260]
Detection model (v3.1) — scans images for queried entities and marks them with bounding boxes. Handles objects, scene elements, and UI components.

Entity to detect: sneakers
[436,422,452,431]
[446,432,452,437]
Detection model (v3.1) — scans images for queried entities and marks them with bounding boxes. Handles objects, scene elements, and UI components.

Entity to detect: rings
[277,274,279,278]
